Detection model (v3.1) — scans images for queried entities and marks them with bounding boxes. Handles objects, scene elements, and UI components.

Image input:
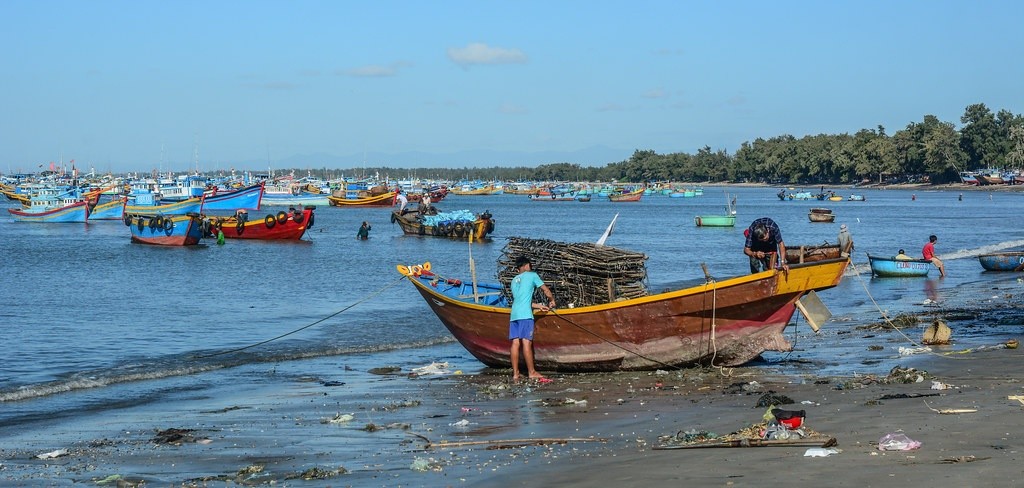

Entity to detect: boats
[808,212,836,222]
[810,208,832,214]
[0,145,450,219]
[529,185,581,200]
[125,213,206,246]
[642,182,703,198]
[608,188,644,202]
[578,194,591,202]
[867,253,932,277]
[695,193,737,226]
[451,172,641,197]
[777,185,865,201]
[979,252,1024,272]
[391,209,495,239]
[204,205,316,240]
[785,242,854,263]
[960,163,1024,185]
[8,184,118,223]
[396,258,850,370]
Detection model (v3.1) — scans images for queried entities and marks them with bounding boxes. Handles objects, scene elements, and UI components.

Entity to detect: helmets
[771,408,806,430]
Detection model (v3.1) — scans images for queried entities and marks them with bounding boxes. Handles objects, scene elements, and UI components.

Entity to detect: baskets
[923,319,951,343]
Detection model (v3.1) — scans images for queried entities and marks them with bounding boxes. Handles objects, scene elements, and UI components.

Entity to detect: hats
[424,193,428,196]
[840,224,848,232]
[362,221,367,226]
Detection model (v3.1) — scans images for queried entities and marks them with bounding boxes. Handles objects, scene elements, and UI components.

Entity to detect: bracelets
[780,260,785,263]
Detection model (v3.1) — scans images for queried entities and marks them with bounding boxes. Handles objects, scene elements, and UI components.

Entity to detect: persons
[922,234,945,278]
[356,221,372,240]
[912,194,916,201]
[836,224,855,260]
[744,217,789,275]
[422,193,431,214]
[508,256,556,379]
[895,249,914,261]
[215,226,225,245]
[395,193,408,215]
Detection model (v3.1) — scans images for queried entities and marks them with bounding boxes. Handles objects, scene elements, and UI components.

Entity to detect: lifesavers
[391,213,396,223]
[186,212,199,217]
[138,219,145,226]
[419,225,425,235]
[265,214,276,226]
[304,205,316,210]
[148,218,155,228]
[125,217,132,226]
[155,216,163,228]
[162,219,172,230]
[236,222,244,231]
[782,194,824,200]
[277,211,288,223]
[310,215,314,226]
[307,221,311,229]
[528,191,574,199]
[429,206,437,216]
[292,210,304,222]
[431,214,495,236]
[407,192,441,201]
